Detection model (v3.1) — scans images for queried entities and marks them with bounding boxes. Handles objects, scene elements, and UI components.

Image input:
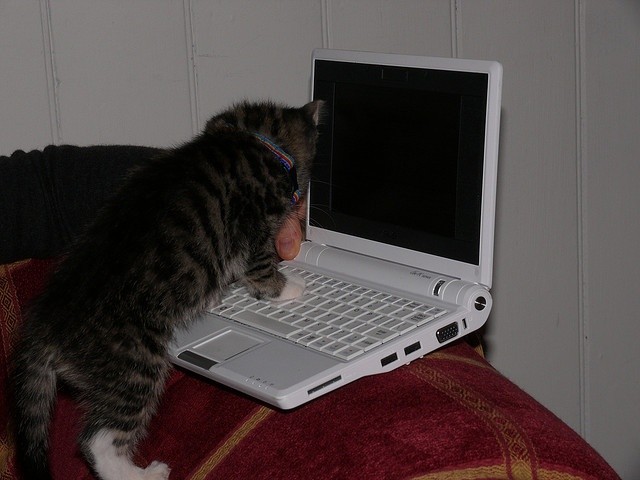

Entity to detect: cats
[6,98,326,480]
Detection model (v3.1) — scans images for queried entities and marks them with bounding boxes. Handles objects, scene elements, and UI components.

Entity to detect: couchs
[0,256,623,480]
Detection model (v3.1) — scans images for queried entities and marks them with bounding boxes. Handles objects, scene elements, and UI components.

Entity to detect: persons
[0,145,308,265]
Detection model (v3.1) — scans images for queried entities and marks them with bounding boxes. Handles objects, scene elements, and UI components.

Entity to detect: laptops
[167,48,504,414]
